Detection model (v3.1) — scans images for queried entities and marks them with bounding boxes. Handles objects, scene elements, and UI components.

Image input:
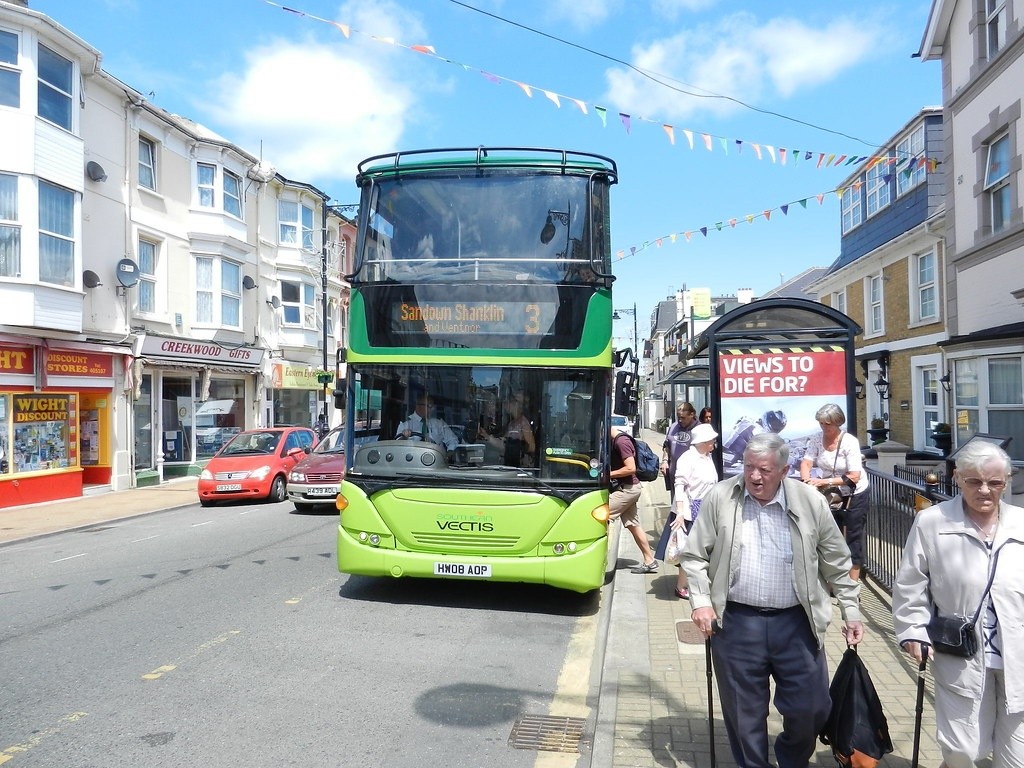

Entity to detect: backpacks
[819,640,894,768]
[614,429,660,482]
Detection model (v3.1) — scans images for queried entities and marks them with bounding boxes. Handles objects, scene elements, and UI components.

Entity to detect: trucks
[194,400,240,450]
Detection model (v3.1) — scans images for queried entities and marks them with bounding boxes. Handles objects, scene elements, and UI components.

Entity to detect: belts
[725,600,802,616]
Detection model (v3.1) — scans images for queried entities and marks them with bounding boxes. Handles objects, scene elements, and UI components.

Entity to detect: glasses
[957,470,1009,489]
[417,403,434,408]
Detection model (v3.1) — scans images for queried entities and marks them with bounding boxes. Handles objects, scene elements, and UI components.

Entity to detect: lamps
[854,378,866,399]
[874,376,892,400]
[940,371,952,393]
[881,276,887,280]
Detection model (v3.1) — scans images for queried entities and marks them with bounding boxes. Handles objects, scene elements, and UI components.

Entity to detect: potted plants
[929,422,951,450]
[866,417,890,445]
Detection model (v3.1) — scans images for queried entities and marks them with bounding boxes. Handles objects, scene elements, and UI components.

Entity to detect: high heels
[675,587,690,600]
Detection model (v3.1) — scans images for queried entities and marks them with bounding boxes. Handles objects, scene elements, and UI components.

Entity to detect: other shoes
[631,560,660,573]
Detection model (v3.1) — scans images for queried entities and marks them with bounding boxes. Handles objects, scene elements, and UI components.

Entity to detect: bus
[332,143,641,595]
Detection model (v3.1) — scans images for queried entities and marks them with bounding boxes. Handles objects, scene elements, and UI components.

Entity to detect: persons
[313,414,324,440]
[479,391,536,467]
[800,403,869,581]
[670,424,719,600]
[679,433,869,768]
[699,407,712,423]
[660,402,700,504]
[892,440,1024,768]
[611,428,659,573]
[396,394,460,459]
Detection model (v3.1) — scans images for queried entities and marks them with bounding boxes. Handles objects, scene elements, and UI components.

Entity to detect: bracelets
[662,459,668,461]
[828,478,832,486]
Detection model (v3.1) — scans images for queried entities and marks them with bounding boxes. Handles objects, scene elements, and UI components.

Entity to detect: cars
[285,420,381,514]
[611,414,631,439]
[197,425,321,508]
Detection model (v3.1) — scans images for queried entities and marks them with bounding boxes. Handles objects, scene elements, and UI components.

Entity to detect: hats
[690,423,718,444]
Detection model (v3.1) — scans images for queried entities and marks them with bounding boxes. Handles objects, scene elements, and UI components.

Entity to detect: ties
[421,418,429,442]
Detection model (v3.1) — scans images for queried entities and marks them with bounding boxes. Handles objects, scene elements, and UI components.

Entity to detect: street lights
[321,196,372,451]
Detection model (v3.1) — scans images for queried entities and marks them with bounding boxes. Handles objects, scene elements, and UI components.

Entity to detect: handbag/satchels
[690,499,702,523]
[664,526,689,565]
[928,615,977,658]
[816,474,843,511]
